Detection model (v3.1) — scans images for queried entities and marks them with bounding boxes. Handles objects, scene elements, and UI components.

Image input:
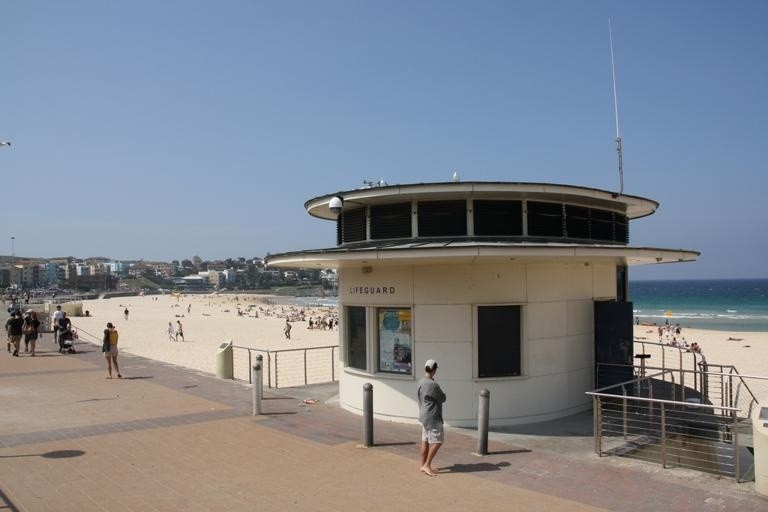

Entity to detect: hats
[10,310,20,316]
[26,309,32,313]
[425,359,438,371]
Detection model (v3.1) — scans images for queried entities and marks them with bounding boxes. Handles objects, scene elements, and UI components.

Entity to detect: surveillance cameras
[327,197,343,214]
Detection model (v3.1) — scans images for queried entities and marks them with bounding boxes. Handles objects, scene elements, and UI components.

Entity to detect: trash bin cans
[751,400,768,497]
[215,339,233,379]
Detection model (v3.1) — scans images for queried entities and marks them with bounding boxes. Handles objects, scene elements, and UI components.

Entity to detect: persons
[123,308,129,320]
[0,290,31,315]
[4,311,16,353]
[57,312,71,353]
[102,322,123,380]
[307,315,340,333]
[26,311,43,356]
[634,316,699,353]
[175,320,184,342]
[167,321,175,342]
[52,305,64,343]
[9,310,23,357]
[414,359,446,479]
[283,321,291,340]
[23,308,32,352]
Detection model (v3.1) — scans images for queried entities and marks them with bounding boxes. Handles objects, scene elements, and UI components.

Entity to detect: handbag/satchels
[23,326,29,334]
[27,326,34,334]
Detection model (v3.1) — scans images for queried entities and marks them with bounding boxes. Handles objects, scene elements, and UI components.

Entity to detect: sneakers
[107,373,122,379]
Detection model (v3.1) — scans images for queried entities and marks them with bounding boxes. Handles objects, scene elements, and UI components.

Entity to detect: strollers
[59,327,76,354]
